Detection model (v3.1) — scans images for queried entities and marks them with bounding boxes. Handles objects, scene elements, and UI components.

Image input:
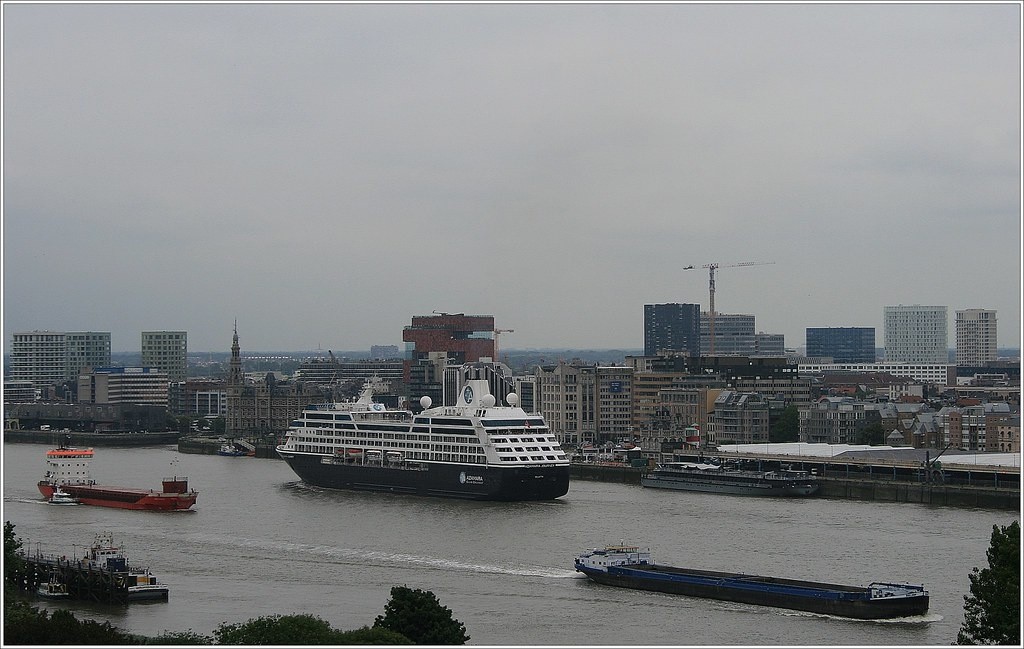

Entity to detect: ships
[274,380,570,501]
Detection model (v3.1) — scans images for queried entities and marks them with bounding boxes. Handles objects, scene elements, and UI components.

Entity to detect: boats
[347,448,363,457]
[48,488,81,506]
[386,452,403,463]
[367,450,382,461]
[37,432,200,512]
[214,441,255,457]
[573,539,930,621]
[22,530,169,603]
[640,467,824,496]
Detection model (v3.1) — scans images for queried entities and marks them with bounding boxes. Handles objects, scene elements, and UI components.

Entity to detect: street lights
[72,544,79,565]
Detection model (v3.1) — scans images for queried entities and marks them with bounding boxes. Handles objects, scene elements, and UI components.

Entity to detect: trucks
[40,425,51,430]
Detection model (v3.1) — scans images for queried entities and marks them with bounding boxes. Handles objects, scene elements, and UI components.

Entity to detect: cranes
[493,328,515,363]
[683,259,776,355]
